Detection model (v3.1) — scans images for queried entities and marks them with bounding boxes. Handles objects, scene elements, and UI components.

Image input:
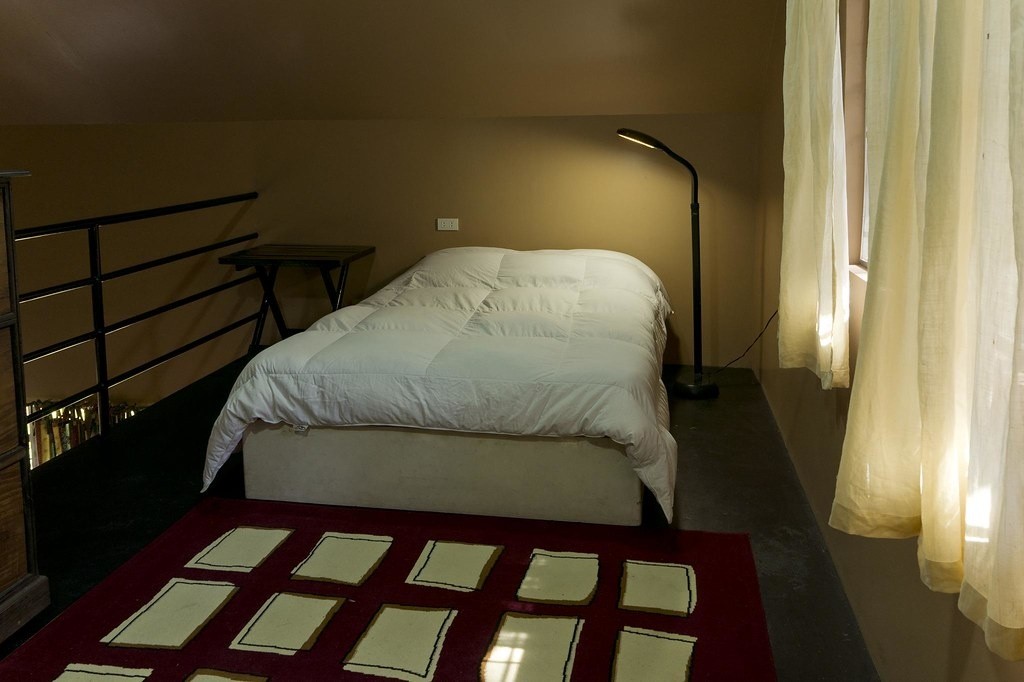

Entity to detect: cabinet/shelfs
[0,174,51,648]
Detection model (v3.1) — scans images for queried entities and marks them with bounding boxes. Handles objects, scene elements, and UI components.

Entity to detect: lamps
[616,127,724,400]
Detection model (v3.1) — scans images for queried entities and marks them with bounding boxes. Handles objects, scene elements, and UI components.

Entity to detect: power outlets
[438,218,459,231]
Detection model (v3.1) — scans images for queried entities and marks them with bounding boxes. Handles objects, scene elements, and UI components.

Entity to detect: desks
[216,245,375,362]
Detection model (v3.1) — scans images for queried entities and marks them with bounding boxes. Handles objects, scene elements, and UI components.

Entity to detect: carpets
[0,497,779,682]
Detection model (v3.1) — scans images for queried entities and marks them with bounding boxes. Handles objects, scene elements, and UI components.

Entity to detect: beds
[201,246,679,527]
